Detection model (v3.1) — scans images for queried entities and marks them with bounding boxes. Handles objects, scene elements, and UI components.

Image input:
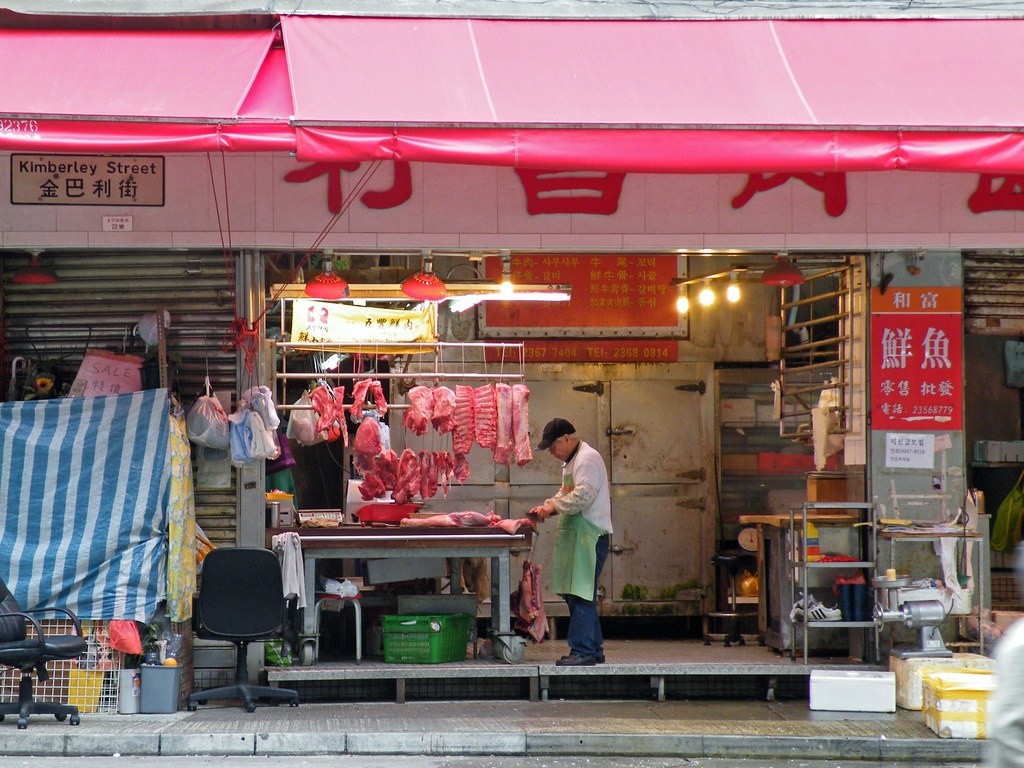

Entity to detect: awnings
[0,3,1024,176]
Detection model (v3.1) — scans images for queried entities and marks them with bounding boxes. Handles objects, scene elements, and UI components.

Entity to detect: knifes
[526,510,557,523]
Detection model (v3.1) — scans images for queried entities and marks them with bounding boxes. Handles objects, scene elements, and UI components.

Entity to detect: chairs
[186,546,299,712]
[0,577,87,729]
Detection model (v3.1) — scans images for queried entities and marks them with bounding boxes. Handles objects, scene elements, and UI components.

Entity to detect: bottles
[801,522,821,562]
[145,644,159,664]
[414,578,431,595]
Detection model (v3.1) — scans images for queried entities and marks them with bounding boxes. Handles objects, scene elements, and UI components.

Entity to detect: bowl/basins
[408,512,445,519]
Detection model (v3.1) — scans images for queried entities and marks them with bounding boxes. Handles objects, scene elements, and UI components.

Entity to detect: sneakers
[795,602,843,621]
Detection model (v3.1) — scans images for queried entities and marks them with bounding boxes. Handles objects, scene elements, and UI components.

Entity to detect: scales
[298,509,343,525]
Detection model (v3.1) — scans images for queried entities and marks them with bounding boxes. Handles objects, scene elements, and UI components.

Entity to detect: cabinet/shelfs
[788,501,881,665]
[870,529,983,662]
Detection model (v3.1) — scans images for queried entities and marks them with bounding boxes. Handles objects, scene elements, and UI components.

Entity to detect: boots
[839,584,855,622]
[855,585,874,622]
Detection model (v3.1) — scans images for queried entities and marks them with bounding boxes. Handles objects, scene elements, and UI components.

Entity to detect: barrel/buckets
[836,583,869,622]
[366,619,385,655]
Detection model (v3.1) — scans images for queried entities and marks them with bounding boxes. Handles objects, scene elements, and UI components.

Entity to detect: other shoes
[556,653,596,665]
[596,655,605,663]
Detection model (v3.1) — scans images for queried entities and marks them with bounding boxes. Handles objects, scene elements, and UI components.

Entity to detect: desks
[265,526,532,665]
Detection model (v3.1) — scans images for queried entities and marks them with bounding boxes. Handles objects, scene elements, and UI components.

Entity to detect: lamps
[401,257,446,300]
[12,252,58,283]
[760,252,804,286]
[306,250,350,299]
[269,284,571,301]
[670,265,748,314]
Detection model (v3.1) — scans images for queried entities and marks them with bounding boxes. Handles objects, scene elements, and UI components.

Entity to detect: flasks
[266,502,280,529]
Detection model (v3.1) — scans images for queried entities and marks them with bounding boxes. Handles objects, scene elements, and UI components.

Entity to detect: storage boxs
[809,670,897,712]
[380,613,469,664]
[973,440,1024,463]
[922,667,995,738]
[889,653,993,710]
[990,541,1024,632]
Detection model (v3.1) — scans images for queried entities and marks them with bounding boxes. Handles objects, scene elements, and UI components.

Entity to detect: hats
[538,418,576,450]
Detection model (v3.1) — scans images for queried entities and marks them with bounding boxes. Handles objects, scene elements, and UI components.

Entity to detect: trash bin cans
[140,663,183,714]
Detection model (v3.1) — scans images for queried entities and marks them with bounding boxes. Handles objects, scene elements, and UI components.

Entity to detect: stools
[703,552,759,647]
[313,593,363,664]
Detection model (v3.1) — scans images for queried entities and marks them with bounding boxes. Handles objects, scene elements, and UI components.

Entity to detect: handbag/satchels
[991,472,1024,552]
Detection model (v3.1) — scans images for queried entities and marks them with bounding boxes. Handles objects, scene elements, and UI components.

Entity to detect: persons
[530,417,614,666]
